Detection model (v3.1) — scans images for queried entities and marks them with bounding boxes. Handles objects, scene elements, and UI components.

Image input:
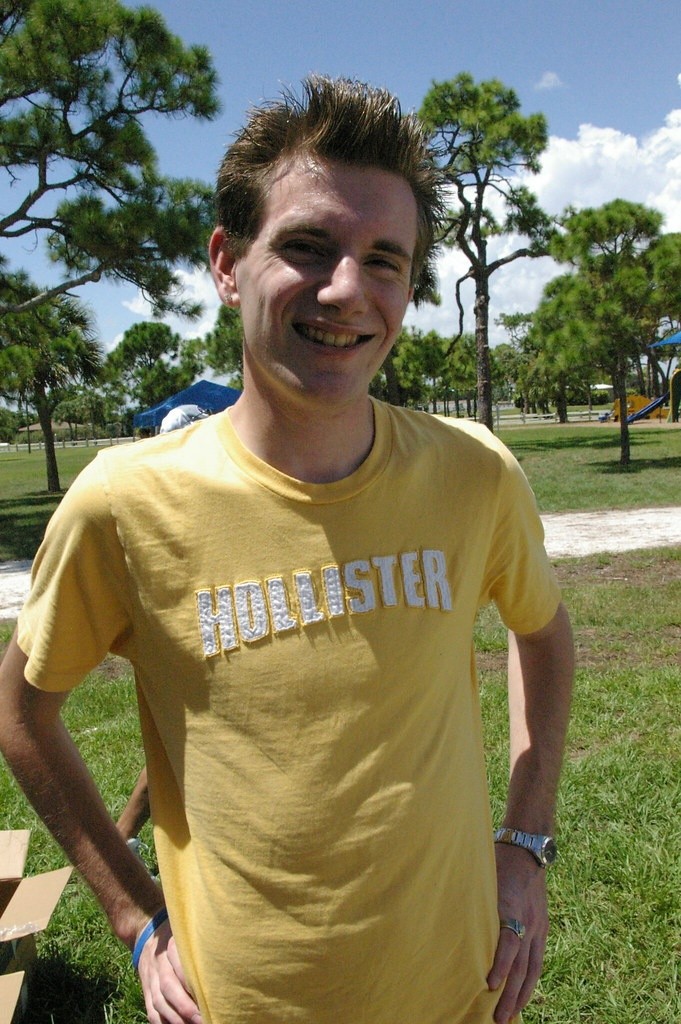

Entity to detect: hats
[159,404,212,434]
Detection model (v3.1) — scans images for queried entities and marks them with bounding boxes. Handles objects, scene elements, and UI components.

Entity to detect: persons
[114,400,205,848]
[0,82,578,1024]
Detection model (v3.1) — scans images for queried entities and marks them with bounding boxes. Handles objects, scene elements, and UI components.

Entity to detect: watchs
[494,827,561,868]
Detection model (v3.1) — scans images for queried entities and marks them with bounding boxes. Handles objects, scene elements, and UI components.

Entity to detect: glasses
[192,408,214,420]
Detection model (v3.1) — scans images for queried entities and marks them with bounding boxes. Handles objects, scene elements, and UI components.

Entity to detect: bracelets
[131,907,168,970]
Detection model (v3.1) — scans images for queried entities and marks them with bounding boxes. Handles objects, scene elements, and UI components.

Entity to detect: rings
[498,918,527,940]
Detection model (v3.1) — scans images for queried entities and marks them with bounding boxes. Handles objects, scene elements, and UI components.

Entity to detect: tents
[133,380,244,437]
